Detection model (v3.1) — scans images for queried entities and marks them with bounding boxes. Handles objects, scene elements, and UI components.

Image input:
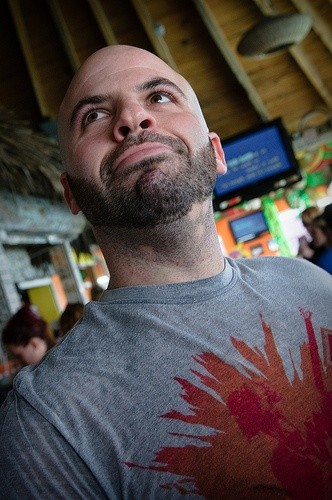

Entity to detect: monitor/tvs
[229,209,269,243]
[213,117,301,212]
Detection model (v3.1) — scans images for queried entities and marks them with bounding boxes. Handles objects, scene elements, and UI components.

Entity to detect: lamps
[237,1,312,55]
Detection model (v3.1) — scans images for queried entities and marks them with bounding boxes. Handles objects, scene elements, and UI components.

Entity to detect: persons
[0,205,331,401]
[0,45,332,500]
